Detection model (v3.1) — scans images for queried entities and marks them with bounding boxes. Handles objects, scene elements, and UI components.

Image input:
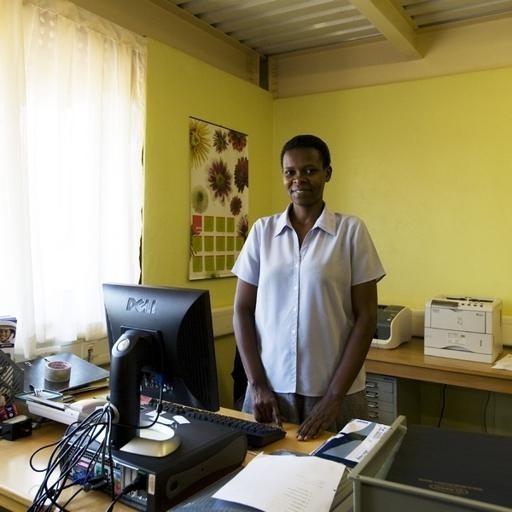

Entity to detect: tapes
[44,361,72,383]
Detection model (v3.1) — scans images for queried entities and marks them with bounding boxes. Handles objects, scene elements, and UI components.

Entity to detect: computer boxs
[56,409,250,512]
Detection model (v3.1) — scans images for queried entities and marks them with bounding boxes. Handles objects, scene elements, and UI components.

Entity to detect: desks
[1,348,368,512]
[345,328,512,428]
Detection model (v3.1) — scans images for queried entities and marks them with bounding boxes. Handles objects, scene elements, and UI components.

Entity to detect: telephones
[26,395,110,428]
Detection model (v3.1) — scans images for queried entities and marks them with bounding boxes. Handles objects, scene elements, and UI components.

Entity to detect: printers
[368,303,413,350]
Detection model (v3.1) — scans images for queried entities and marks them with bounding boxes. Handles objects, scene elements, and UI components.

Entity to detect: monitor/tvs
[84,281,222,458]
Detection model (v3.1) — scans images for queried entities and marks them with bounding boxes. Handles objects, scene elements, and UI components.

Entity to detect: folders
[15,351,111,392]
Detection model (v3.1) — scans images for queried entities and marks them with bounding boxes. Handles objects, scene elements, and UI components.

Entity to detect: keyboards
[142,396,287,450]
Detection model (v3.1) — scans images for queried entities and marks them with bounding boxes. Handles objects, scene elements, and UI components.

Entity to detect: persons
[232,134,386,440]
[1,321,15,343]
